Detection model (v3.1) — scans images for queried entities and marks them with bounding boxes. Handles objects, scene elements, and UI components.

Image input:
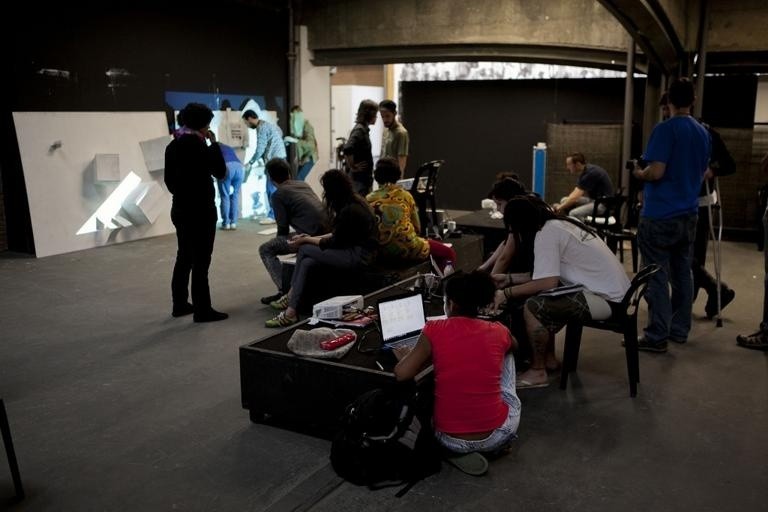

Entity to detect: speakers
[533,147,547,201]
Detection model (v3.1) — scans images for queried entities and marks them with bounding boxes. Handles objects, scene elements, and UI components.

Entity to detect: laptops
[430,254,445,280]
[376,290,426,356]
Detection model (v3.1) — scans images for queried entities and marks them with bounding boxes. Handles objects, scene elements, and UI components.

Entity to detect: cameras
[626,155,647,169]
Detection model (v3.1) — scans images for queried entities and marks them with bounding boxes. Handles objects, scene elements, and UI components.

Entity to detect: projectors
[481,199,498,209]
[397,176,428,190]
[312,296,365,319]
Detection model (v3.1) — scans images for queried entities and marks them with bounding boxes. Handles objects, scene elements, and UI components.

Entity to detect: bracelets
[506,273,512,286]
[503,286,512,300]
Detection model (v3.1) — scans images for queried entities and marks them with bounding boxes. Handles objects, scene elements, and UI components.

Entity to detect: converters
[448,221,456,232]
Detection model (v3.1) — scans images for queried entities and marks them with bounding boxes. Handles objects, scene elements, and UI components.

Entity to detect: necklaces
[669,112,692,117]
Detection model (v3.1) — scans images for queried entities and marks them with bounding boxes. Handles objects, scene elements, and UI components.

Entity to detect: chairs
[604,189,638,274]
[410,159,445,236]
[591,188,627,261]
[560,263,663,395]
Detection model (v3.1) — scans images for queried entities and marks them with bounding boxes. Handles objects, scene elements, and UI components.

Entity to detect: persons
[379,100,409,182]
[621,77,709,352]
[285,106,319,182]
[737,140,768,351]
[340,98,378,198]
[392,273,523,476]
[264,168,380,329]
[486,196,635,390]
[477,179,544,371]
[240,110,287,223]
[552,152,614,221]
[213,139,243,231]
[658,93,735,320]
[365,156,457,277]
[164,102,228,324]
[258,158,334,304]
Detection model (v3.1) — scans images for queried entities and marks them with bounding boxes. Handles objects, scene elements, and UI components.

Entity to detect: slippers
[515,374,551,391]
[620,333,669,353]
[526,358,562,373]
[643,325,688,345]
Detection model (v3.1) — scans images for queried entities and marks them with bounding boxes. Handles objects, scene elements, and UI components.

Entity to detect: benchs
[280,255,397,312]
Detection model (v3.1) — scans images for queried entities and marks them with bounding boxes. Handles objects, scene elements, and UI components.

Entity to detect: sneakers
[270,295,291,309]
[219,223,230,230]
[704,282,735,320]
[736,323,768,350]
[230,223,237,230]
[447,450,489,476]
[264,311,299,328]
[171,303,194,317]
[193,309,229,324]
[260,293,285,305]
[259,216,276,225]
